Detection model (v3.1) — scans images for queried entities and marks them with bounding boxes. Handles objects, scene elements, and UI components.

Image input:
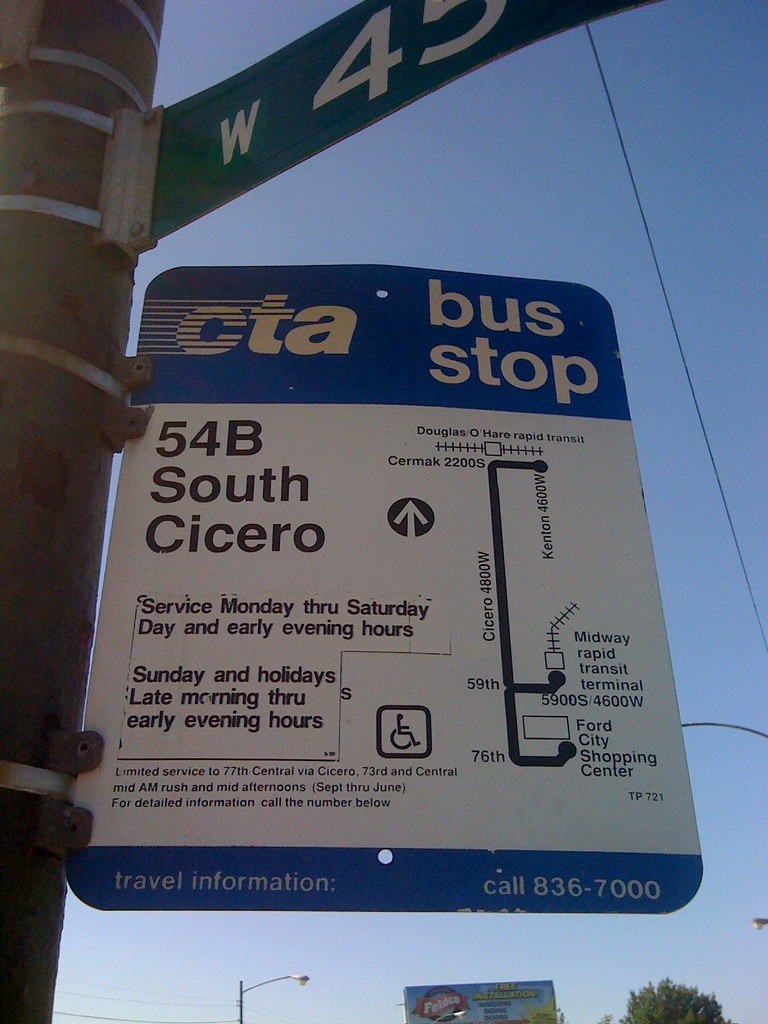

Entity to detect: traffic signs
[152,0,662,239]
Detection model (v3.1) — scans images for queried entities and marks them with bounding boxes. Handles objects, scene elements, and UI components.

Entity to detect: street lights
[239,974,308,1023]
